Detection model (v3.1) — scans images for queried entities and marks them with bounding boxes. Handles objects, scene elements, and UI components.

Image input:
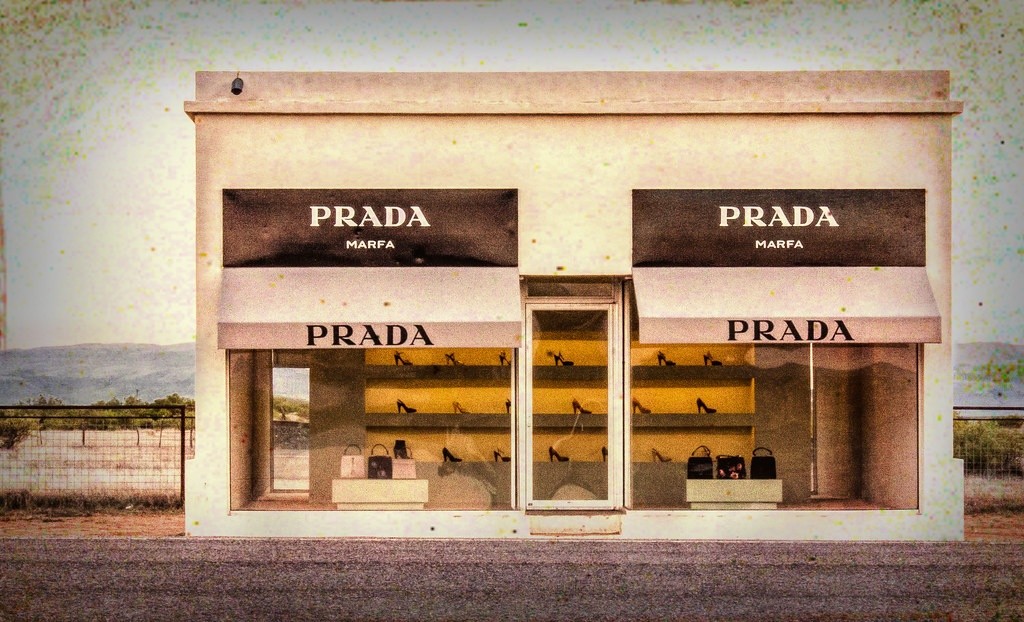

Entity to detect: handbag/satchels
[716,454,747,479]
[368,443,393,480]
[394,446,417,480]
[688,445,713,479]
[340,444,367,479]
[750,447,777,479]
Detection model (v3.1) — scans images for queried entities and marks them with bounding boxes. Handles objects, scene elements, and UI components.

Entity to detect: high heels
[651,447,672,462]
[442,447,462,463]
[505,398,511,413]
[445,352,465,366]
[702,350,723,366]
[499,351,511,366]
[549,446,570,462]
[631,397,652,414]
[393,440,414,459]
[452,401,471,413]
[657,350,676,366]
[397,398,417,413]
[493,446,511,463]
[394,351,413,366]
[553,351,574,366]
[572,398,592,415]
[601,446,608,462]
[696,397,717,413]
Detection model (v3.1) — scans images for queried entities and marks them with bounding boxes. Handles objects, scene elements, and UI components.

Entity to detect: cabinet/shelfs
[364,364,755,507]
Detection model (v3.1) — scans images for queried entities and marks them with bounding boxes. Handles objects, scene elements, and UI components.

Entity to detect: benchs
[331,479,428,511]
[685,479,782,510]
